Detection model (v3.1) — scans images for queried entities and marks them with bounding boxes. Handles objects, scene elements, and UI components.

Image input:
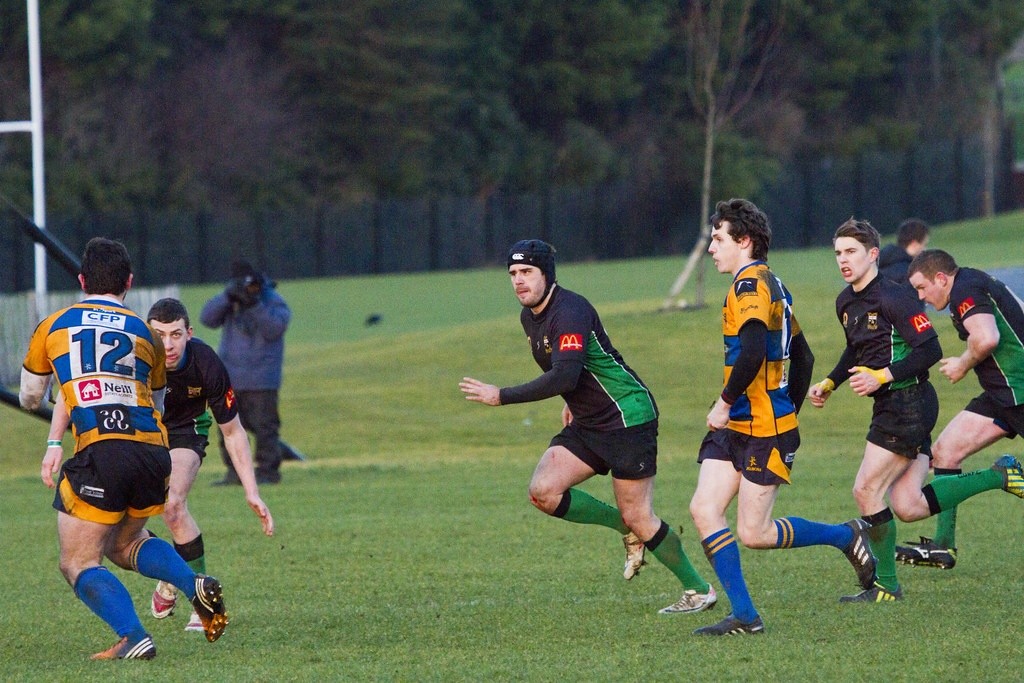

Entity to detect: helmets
[507,240,556,284]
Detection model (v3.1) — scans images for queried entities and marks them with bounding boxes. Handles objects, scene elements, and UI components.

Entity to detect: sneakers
[994,455,1024,499]
[152,573,179,618]
[693,611,763,636]
[90,628,157,660]
[838,518,879,592]
[657,582,716,614]
[622,521,647,580]
[192,575,229,644]
[894,535,958,570]
[840,580,902,604]
[185,606,203,632]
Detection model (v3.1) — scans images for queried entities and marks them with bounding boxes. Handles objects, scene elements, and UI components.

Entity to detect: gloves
[224,278,245,298]
[238,291,260,306]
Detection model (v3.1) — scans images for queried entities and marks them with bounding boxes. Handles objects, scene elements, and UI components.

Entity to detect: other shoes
[211,477,240,486]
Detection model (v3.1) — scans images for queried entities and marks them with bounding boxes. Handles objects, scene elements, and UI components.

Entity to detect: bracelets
[46,440,62,447]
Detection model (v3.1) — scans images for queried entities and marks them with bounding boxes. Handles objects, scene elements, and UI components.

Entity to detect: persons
[20,237,229,659]
[691,199,878,634]
[200,260,290,485]
[875,218,935,473]
[807,217,1024,604]
[901,248,1024,570]
[459,239,717,614]
[41,297,274,631]
